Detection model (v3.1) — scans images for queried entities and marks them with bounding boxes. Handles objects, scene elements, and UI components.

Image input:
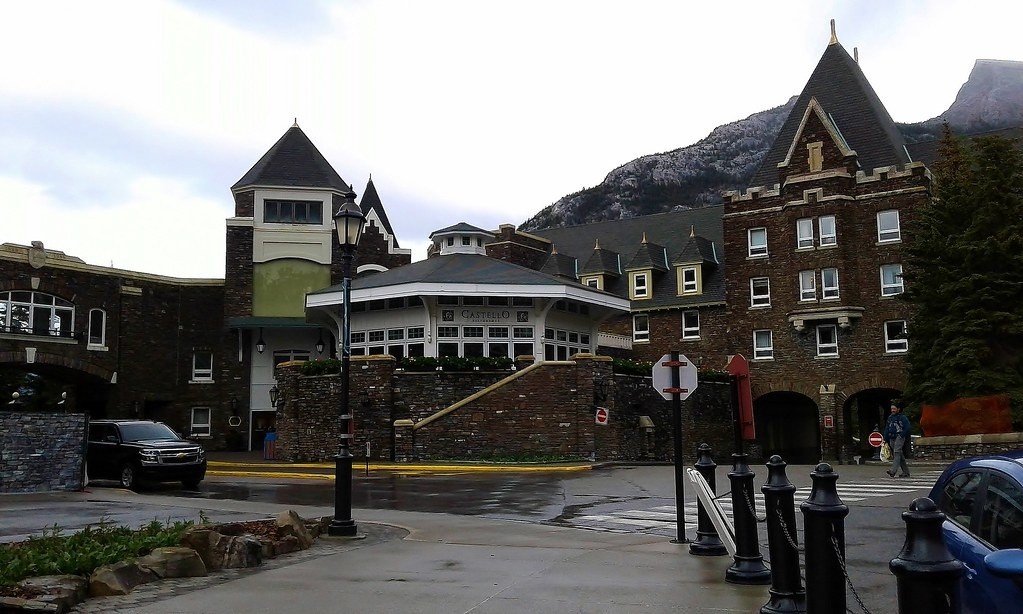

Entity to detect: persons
[883,402,912,478]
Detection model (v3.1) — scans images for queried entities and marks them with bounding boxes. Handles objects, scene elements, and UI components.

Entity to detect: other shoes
[886,469,895,478]
[899,472,911,478]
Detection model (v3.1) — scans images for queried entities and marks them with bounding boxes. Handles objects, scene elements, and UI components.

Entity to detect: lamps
[229,394,241,414]
[268,384,278,407]
[314,327,326,355]
[595,374,610,402]
[360,389,370,405]
[255,327,266,353]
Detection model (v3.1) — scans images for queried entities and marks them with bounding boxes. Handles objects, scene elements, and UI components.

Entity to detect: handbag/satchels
[880,440,890,462]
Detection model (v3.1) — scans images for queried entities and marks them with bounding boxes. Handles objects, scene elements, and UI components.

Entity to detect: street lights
[327,182,368,537]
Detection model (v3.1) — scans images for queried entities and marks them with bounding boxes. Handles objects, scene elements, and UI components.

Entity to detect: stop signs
[597,410,607,423]
[869,432,884,447]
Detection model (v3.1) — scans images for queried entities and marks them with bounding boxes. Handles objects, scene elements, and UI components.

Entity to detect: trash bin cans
[640,416,655,460]
[264,432,276,460]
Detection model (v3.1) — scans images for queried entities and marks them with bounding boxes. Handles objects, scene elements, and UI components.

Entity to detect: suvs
[87,419,208,492]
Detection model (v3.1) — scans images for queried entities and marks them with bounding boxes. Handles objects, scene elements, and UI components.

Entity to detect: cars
[928,450,1023,614]
